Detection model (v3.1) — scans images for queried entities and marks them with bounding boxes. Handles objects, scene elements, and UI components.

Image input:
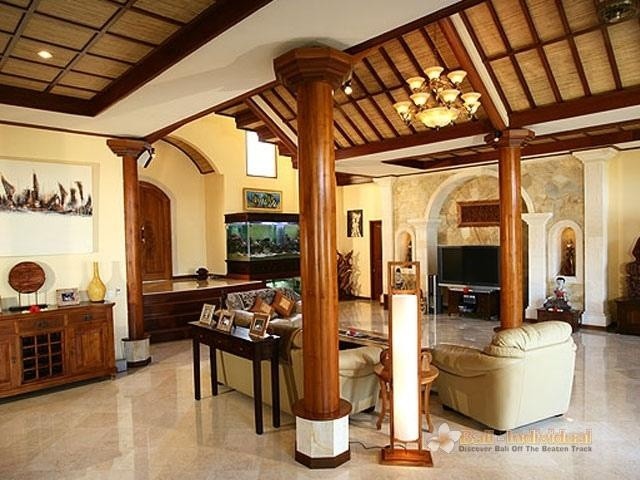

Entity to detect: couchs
[211,287,382,422]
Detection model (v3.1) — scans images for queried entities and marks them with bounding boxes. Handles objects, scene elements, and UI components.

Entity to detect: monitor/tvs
[437,244,501,294]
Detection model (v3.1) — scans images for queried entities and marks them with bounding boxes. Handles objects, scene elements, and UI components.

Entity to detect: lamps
[392,21,481,130]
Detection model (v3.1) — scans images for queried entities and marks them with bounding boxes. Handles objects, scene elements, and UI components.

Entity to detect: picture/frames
[249,312,269,338]
[457,200,500,227]
[199,304,217,325]
[57,288,81,308]
[216,308,235,333]
[243,187,283,211]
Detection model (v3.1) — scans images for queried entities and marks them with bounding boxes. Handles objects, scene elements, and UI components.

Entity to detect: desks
[536,307,585,332]
[447,288,499,320]
[373,361,439,435]
[188,318,281,434]
[0,300,117,403]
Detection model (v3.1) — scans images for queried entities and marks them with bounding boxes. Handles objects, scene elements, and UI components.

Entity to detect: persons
[544,278,569,312]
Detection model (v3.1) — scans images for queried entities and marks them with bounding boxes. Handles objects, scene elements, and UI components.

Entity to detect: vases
[87,262,107,304]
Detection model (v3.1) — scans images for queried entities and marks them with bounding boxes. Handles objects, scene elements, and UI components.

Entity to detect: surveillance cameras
[138,149,157,170]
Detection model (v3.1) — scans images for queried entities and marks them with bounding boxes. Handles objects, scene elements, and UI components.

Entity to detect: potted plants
[614,237,640,334]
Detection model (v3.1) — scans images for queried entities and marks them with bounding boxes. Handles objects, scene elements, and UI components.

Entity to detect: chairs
[432,319,577,436]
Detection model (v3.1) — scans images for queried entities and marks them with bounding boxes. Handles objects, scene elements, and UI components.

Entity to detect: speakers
[427,274,443,314]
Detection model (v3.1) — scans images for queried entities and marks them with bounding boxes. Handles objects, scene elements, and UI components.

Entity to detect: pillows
[250,295,275,316]
[273,293,295,317]
